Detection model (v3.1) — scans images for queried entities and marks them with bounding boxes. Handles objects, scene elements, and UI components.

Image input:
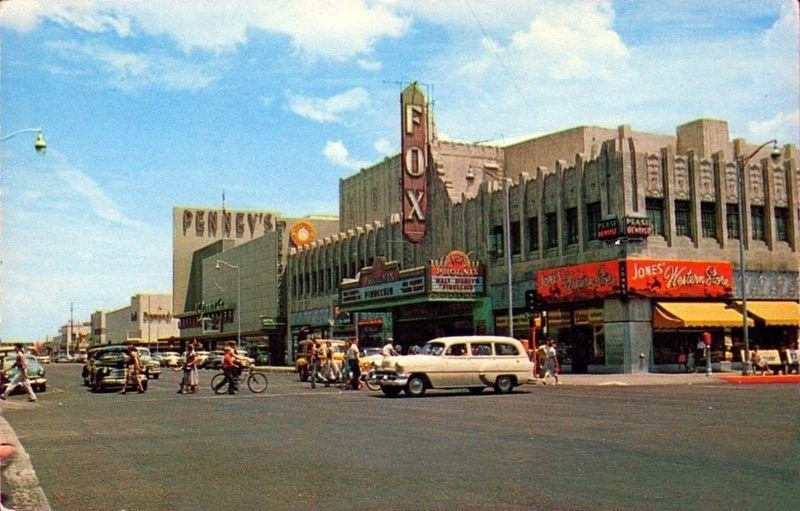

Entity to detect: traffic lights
[525,290,541,328]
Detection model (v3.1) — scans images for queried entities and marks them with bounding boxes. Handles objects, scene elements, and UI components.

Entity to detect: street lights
[0,126,46,158]
[466,164,513,335]
[214,259,240,344]
[735,139,782,375]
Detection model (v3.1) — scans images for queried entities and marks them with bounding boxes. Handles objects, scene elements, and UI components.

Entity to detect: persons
[214,340,249,395]
[752,345,774,376]
[383,338,402,357]
[172,338,200,394]
[307,337,361,390]
[535,337,559,386]
[686,336,706,373]
[118,345,146,394]
[0,343,38,402]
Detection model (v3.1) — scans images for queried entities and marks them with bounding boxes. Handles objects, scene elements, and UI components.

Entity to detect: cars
[0,354,50,396]
[295,335,537,398]
[55,345,256,393]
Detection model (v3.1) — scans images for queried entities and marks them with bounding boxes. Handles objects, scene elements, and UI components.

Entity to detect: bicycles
[210,359,268,394]
